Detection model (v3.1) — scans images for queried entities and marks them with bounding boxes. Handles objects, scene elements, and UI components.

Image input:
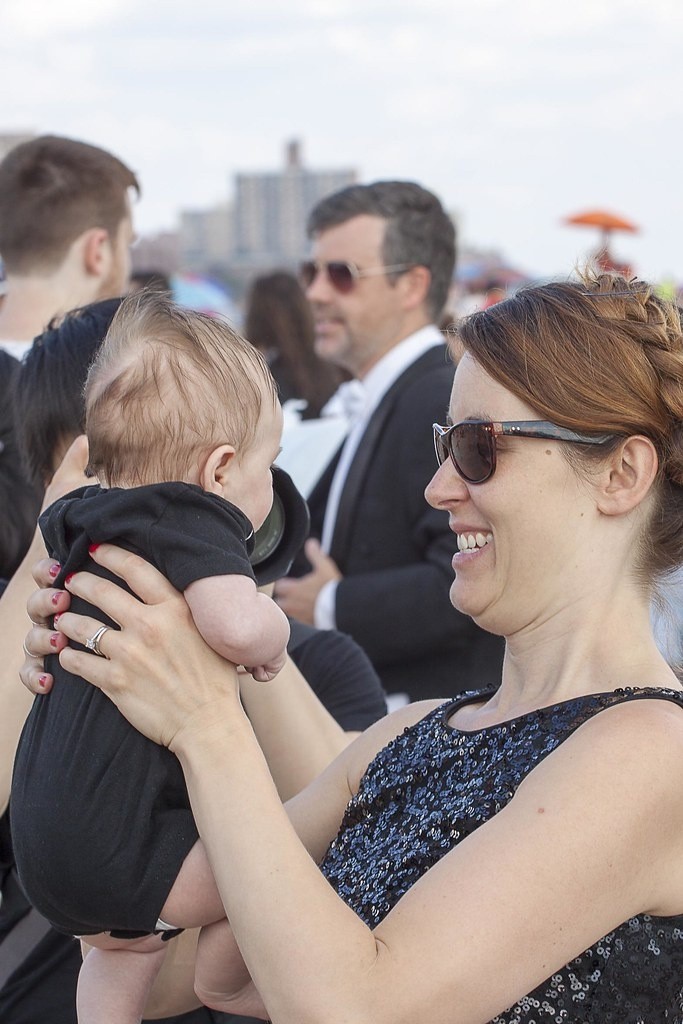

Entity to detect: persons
[0,136,141,587]
[24,269,682,1023]
[268,179,504,696]
[9,292,291,1023]
[1,267,383,1024]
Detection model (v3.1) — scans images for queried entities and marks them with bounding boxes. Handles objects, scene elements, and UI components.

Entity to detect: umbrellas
[564,210,635,246]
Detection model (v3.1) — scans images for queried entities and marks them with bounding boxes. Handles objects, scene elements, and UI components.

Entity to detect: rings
[27,610,47,625]
[86,626,113,658]
[23,644,39,658]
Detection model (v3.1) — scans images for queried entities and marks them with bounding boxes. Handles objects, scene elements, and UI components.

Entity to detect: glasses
[297,260,417,295]
[432,420,620,484]
